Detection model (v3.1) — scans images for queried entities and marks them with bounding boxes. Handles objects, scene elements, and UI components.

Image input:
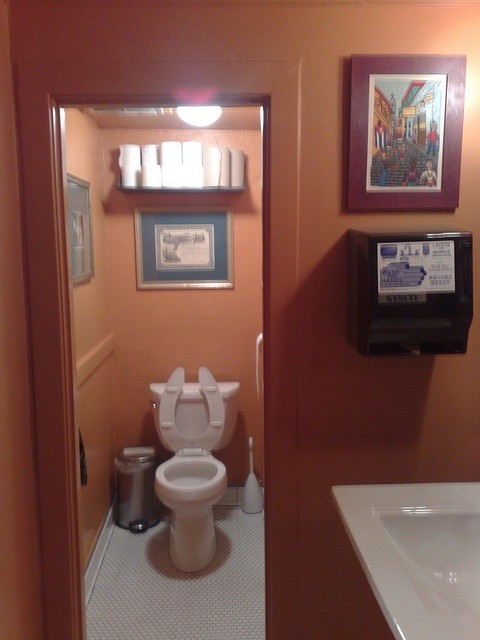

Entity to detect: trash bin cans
[114,448,159,533]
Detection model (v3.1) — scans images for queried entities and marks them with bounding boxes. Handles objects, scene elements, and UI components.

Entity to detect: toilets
[145,364,242,573]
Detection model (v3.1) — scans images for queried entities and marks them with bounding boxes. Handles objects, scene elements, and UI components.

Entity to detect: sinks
[369,501,479,600]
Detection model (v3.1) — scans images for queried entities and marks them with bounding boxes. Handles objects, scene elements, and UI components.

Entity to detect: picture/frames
[347,53,466,212]
[133,208,235,291]
[64,172,91,284]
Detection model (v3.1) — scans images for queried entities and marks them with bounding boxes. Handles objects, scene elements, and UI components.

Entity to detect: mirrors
[16,62,302,636]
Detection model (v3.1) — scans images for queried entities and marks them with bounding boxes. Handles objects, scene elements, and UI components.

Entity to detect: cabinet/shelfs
[114,186,241,197]
